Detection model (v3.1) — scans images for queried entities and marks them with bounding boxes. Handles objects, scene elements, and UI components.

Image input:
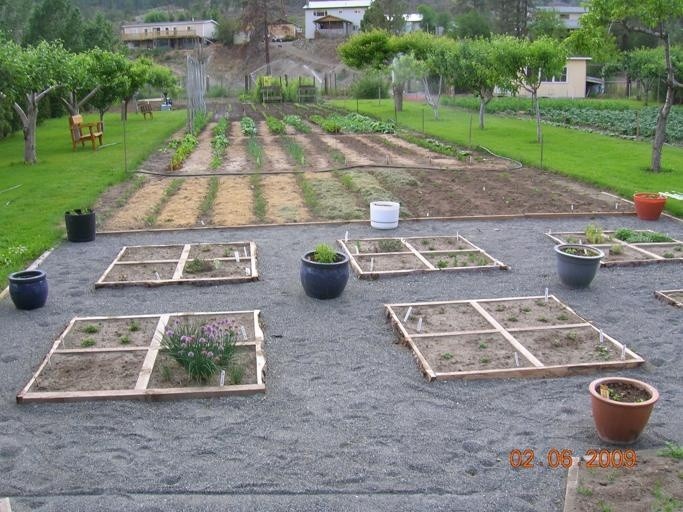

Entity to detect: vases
[5,268,49,310]
[632,190,668,222]
[369,200,400,231]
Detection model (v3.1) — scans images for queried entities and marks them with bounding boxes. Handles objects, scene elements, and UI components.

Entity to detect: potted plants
[552,242,606,291]
[297,241,351,299]
[587,374,660,448]
[63,206,96,242]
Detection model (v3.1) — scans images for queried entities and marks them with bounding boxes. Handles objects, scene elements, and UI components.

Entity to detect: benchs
[138,102,154,120]
[65,112,104,152]
